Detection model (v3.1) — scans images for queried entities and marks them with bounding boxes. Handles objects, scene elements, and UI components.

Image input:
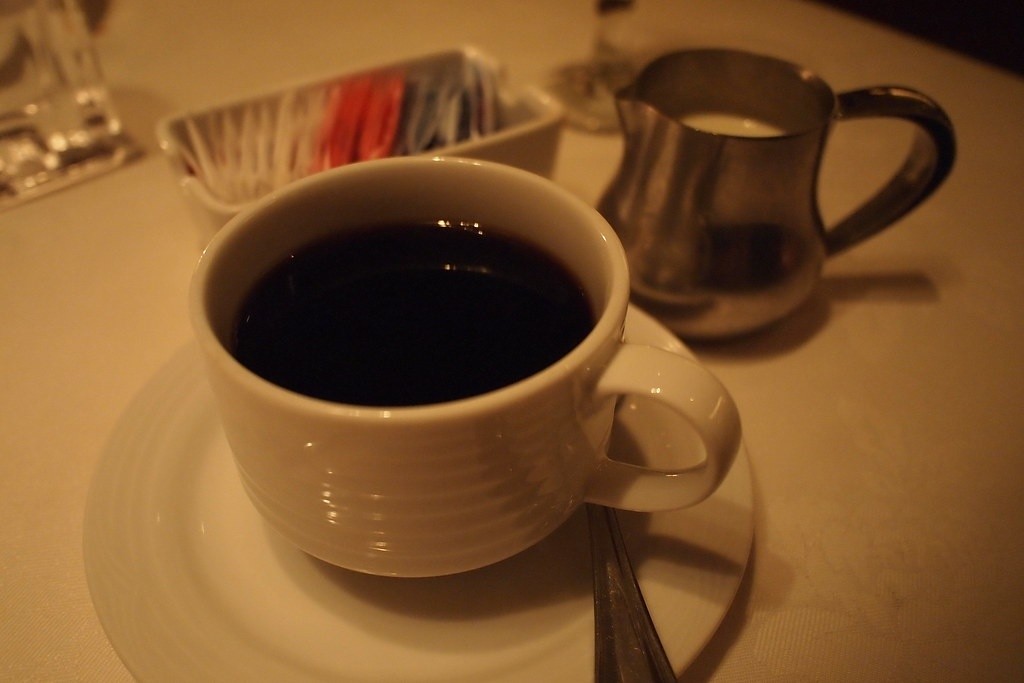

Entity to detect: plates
[82,298,757,683]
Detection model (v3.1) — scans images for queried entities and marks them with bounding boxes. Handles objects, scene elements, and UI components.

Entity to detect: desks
[0,0,1024,683]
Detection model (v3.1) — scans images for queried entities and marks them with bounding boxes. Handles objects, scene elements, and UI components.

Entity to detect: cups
[189,154,744,575]
[0,0,127,205]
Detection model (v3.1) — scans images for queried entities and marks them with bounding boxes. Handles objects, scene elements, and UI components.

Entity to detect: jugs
[592,47,958,347]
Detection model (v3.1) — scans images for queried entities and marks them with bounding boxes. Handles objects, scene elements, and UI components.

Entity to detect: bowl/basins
[156,46,568,247]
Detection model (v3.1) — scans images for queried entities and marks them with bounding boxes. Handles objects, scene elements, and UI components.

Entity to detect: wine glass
[541,0,642,135]
